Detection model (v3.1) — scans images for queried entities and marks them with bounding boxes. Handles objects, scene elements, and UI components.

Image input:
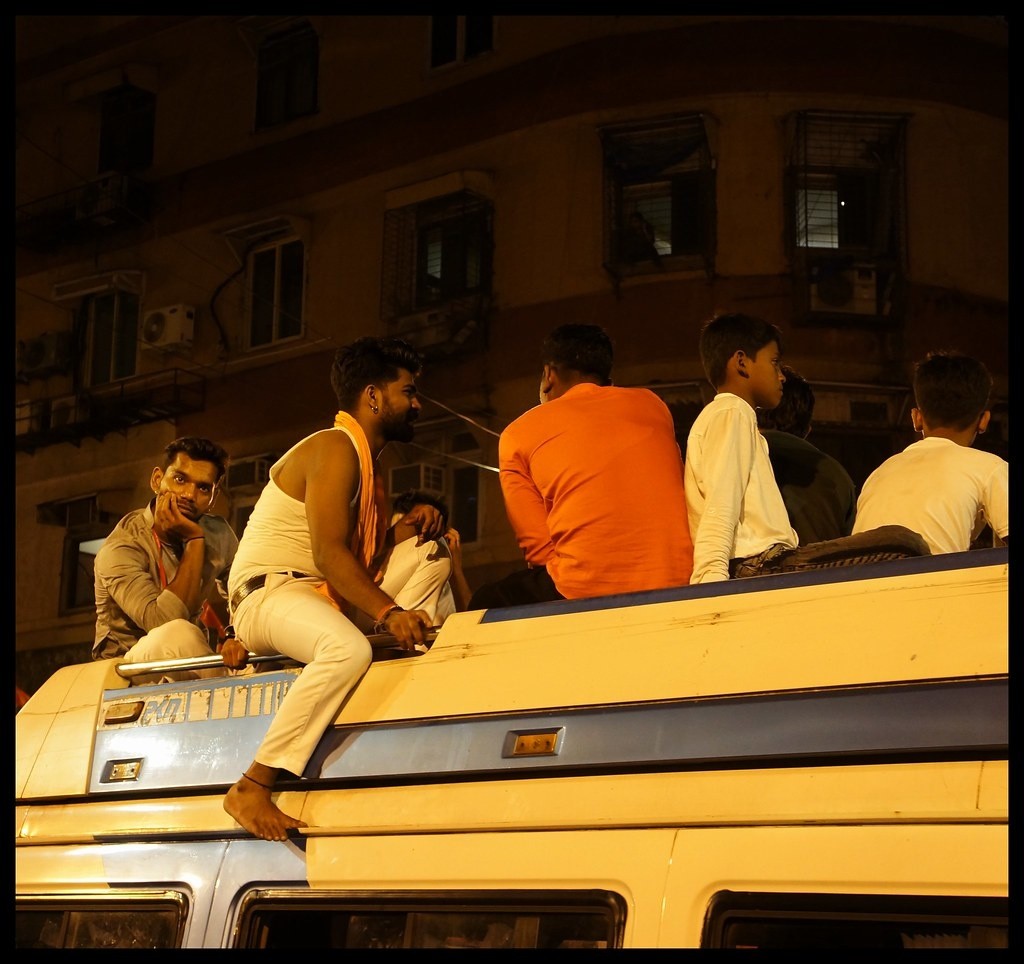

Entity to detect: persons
[390,489,472,612]
[468,323,693,610]
[91,437,248,669]
[754,365,856,546]
[224,337,442,842]
[684,313,799,584]
[852,350,1009,556]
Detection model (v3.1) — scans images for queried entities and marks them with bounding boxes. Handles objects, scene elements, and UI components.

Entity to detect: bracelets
[374,605,405,634]
[185,536,205,543]
[222,634,235,644]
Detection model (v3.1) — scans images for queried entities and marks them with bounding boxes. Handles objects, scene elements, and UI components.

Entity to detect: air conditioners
[75,169,124,221]
[224,457,274,497]
[65,500,96,530]
[387,462,447,497]
[140,303,195,350]
[19,332,71,372]
[809,264,877,317]
